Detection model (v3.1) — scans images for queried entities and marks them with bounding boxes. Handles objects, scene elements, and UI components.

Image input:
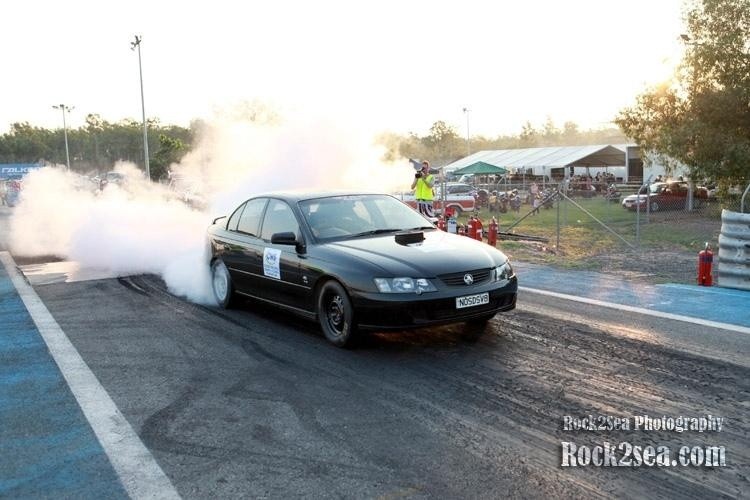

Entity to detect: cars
[387,182,480,218]
[622,181,709,213]
[204,185,520,352]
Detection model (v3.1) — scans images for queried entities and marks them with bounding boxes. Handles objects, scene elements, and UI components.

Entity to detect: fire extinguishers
[458,223,465,236]
[468,215,483,241]
[437,215,447,232]
[696,242,713,287]
[487,216,498,248]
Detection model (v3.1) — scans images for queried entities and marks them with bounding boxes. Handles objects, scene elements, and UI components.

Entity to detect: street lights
[462,107,471,155]
[51,104,76,172]
[681,33,699,211]
[129,32,151,180]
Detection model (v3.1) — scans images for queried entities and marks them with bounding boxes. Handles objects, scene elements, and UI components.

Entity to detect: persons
[94,179,109,194]
[412,161,435,222]
[655,175,683,182]
[486,171,621,216]
